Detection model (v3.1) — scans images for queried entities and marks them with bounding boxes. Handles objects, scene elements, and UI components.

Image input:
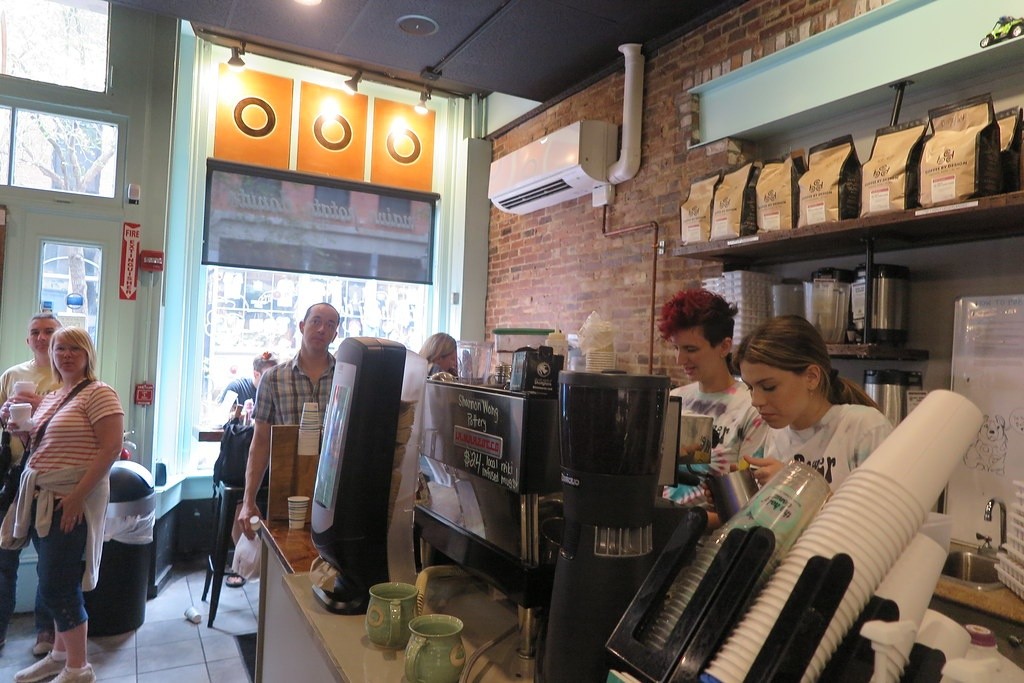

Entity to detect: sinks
[941,552,1001,584]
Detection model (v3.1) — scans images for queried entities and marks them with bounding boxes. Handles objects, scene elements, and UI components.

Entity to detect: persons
[0,312,125,683]
[213,302,341,589]
[419,332,458,376]
[655,289,895,536]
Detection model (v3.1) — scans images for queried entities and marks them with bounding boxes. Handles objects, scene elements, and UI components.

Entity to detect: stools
[202,480,269,628]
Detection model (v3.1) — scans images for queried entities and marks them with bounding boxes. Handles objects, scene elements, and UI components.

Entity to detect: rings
[74,517,75,520]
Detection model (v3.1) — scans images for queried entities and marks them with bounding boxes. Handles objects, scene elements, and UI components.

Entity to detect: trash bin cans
[83,460,156,636]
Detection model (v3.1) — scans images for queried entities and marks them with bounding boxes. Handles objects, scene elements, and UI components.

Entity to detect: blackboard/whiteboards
[941,292,1024,552]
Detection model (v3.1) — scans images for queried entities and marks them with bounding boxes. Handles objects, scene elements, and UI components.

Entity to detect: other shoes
[33,628,55,654]
[0,637,6,646]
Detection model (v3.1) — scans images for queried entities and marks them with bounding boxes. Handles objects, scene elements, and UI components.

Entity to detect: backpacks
[213,424,269,487]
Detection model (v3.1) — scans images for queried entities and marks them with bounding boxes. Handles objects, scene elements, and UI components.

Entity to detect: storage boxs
[491,328,555,352]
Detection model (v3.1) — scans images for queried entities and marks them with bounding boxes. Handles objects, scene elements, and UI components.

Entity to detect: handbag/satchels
[0,502,32,550]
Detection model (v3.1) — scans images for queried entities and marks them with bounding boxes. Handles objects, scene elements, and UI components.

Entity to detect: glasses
[53,343,85,354]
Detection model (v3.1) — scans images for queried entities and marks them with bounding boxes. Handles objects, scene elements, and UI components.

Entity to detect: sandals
[226,575,246,586]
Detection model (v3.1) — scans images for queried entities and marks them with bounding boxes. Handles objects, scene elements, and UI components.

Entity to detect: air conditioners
[488,120,617,216]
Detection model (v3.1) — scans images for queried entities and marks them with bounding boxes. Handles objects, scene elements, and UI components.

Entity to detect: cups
[585,330,615,374]
[183,607,201,624]
[365,582,419,650]
[456,340,494,382]
[404,614,466,683]
[298,402,321,455]
[766,284,803,320]
[802,280,851,344]
[9,403,32,429]
[14,381,36,396]
[641,389,983,683]
[287,496,310,529]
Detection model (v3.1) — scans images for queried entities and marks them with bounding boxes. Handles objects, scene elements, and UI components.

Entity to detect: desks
[191,423,225,442]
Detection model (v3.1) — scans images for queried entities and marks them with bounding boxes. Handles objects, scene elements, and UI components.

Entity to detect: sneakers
[13,649,66,683]
[50,663,96,683]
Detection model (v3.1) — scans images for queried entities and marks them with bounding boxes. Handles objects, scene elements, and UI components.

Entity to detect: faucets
[984,496,1008,554]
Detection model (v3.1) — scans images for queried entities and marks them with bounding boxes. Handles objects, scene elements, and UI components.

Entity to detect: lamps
[414,91,429,115]
[343,71,361,96]
[228,47,246,72]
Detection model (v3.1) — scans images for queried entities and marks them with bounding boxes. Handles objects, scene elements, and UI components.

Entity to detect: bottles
[545,324,567,368]
[494,365,511,386]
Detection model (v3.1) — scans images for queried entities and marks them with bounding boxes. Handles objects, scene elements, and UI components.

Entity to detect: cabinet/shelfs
[673,32,1024,360]
